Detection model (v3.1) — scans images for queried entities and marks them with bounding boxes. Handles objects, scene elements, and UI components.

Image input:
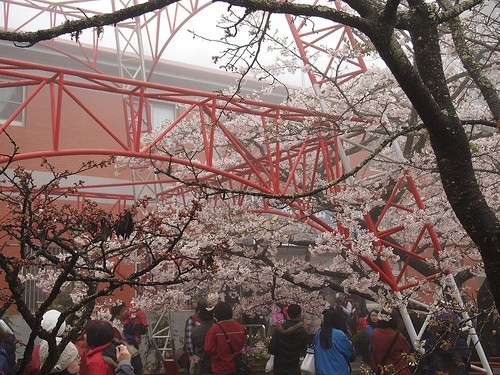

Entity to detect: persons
[0,301,248,375]
[267,304,308,375]
[312,290,500,375]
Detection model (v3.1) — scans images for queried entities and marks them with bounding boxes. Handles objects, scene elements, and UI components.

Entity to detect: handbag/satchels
[233,352,250,375]
[265,355,275,373]
[300,328,322,375]
[350,314,355,327]
[189,354,200,375]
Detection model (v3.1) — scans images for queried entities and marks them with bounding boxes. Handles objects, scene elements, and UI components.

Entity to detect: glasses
[73,354,81,362]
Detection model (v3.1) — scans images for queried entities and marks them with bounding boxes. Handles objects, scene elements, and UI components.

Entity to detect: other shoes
[349,356,356,362]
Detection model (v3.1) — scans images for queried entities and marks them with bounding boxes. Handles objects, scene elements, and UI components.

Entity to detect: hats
[41,309,66,336]
[38,336,79,373]
[205,292,219,312]
